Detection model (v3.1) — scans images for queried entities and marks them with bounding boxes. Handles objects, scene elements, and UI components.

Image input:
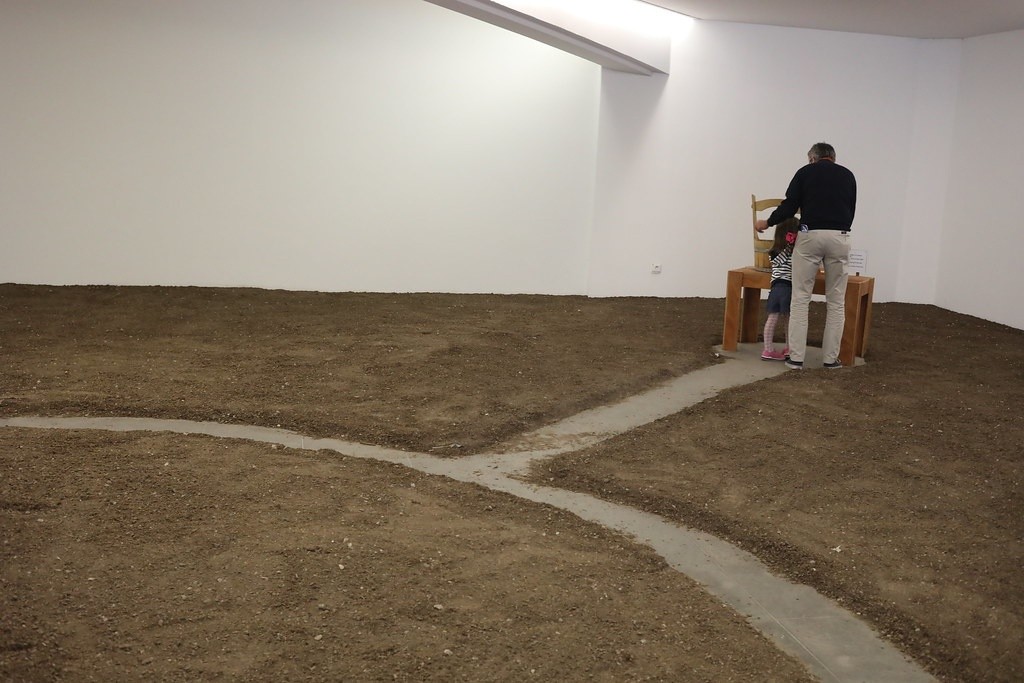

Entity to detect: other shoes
[783,347,790,358]
[823,360,842,369]
[761,350,785,360]
[784,357,802,370]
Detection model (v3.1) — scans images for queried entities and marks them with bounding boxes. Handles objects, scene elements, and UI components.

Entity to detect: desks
[721,266,876,369]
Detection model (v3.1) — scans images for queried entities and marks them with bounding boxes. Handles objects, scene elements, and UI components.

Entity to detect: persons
[755,142,857,370]
[761,218,800,362]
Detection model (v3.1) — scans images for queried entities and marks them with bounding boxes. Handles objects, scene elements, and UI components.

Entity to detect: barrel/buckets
[750,194,783,272]
